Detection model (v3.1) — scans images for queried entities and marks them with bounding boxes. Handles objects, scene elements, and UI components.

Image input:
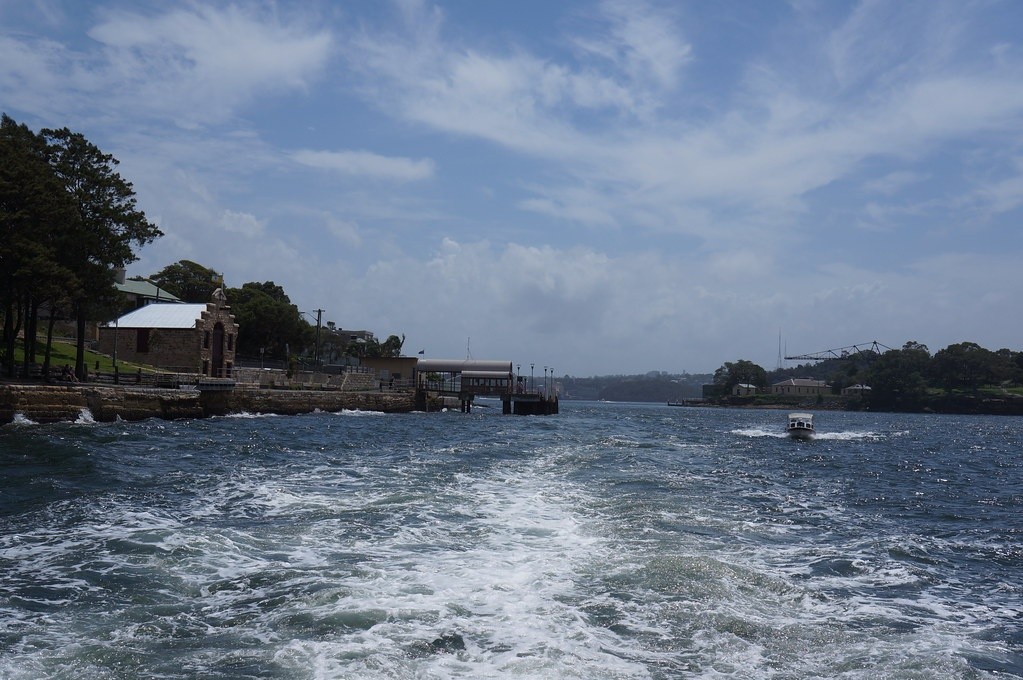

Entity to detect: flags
[211,275,222,283]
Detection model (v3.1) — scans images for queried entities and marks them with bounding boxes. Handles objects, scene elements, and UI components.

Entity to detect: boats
[782,412,818,440]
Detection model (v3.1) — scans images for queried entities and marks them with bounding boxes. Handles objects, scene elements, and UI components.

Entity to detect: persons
[62,364,79,382]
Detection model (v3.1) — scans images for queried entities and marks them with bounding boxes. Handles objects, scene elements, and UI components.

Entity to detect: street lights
[516,363,522,394]
[530,360,535,394]
[550,367,555,394]
[543,364,549,400]
